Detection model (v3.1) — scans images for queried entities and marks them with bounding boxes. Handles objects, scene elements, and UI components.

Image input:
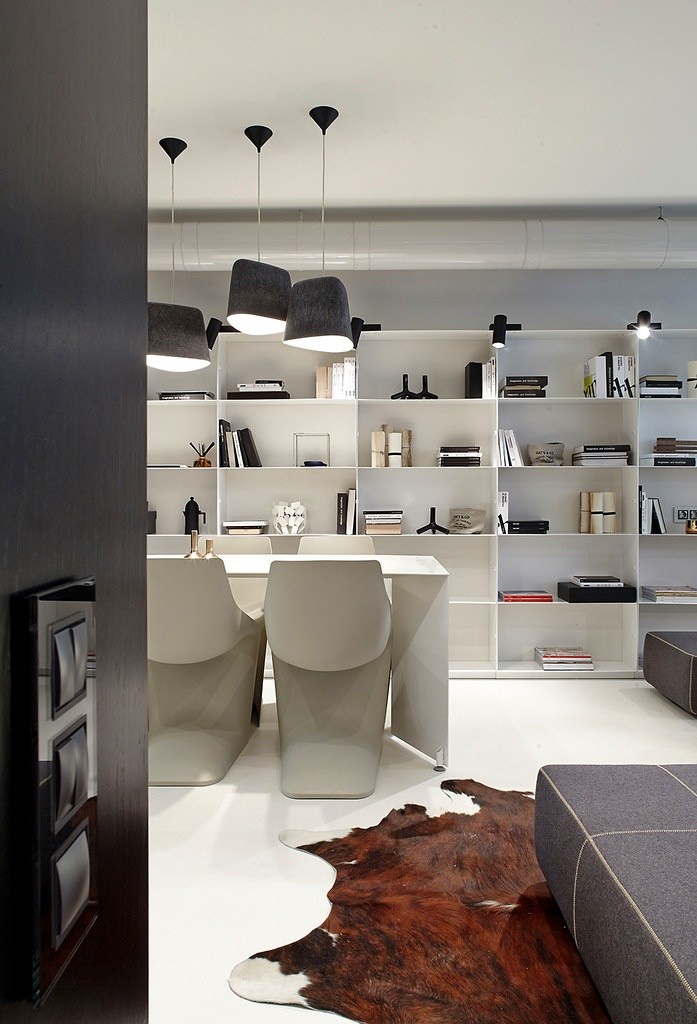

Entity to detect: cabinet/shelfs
[148,327,697,680]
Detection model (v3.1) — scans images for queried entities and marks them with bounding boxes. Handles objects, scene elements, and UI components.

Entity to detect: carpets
[227,777,618,1024]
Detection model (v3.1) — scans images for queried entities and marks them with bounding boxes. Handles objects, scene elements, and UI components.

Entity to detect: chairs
[196,535,272,553]
[147,557,261,785]
[265,559,391,799]
[298,535,376,555]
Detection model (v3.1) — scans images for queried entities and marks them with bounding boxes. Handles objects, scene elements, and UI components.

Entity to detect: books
[499,589,552,602]
[437,446,482,467]
[464,357,548,398]
[641,585,697,602]
[363,511,404,535]
[574,445,630,466]
[315,357,356,400]
[557,576,637,603]
[498,429,525,466]
[534,646,594,671]
[157,391,214,401]
[583,352,635,398]
[639,375,682,398]
[218,419,263,466]
[640,437,696,466]
[337,489,357,535]
[227,379,290,399]
[639,485,667,534]
[222,519,268,534]
[498,492,549,534]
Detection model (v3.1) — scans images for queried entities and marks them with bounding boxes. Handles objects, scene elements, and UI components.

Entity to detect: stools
[643,631,697,715]
[535,764,697,1022]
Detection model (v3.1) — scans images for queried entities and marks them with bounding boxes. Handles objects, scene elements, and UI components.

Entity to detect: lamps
[627,310,664,340]
[349,317,380,350]
[282,105,354,352]
[145,138,209,372]
[226,124,292,332]
[489,315,521,348]
[204,317,221,350]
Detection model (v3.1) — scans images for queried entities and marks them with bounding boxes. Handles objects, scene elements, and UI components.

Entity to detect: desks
[147,553,450,772]
